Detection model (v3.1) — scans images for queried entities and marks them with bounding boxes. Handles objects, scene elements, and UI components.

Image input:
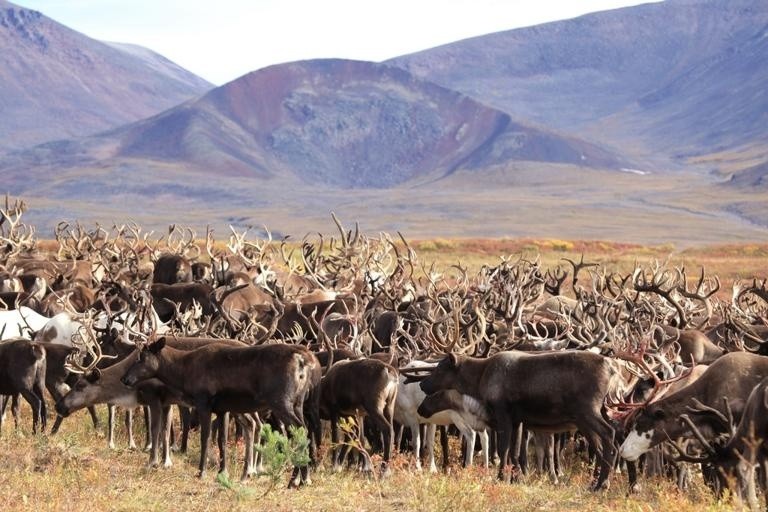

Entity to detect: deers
[0,192,768,512]
